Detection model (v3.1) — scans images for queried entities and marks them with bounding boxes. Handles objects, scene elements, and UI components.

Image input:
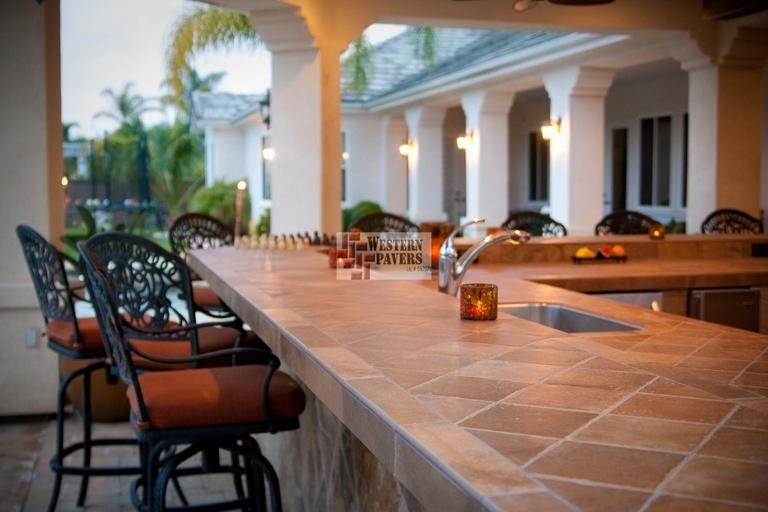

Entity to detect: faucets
[440,218,531,296]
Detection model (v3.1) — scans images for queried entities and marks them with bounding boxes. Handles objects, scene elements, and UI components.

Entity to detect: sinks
[496,304,646,334]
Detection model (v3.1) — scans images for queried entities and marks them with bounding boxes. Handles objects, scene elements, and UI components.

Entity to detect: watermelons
[598,247,612,258]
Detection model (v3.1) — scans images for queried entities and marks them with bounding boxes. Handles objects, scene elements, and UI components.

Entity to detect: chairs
[500,211,567,236]
[594,210,662,235]
[347,211,422,232]
[699,208,762,235]
[13,211,307,512]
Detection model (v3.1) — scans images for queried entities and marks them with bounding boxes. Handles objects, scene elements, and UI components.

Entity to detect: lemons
[576,247,596,258]
